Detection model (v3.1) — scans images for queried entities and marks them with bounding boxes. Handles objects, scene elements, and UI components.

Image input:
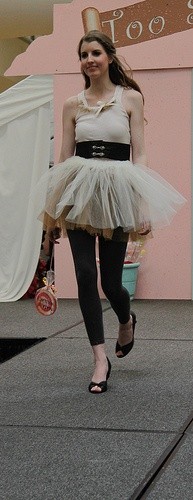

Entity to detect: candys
[35,243,57,317]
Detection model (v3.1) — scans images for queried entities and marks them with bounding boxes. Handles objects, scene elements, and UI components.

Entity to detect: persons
[35,30,187,394]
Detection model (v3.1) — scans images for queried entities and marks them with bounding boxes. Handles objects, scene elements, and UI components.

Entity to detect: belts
[74,140,130,161]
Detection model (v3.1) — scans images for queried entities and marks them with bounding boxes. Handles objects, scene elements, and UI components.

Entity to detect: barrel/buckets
[97,262,140,301]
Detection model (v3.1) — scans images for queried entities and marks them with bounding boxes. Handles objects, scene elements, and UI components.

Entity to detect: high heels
[87,357,111,393]
[114,311,137,359]
